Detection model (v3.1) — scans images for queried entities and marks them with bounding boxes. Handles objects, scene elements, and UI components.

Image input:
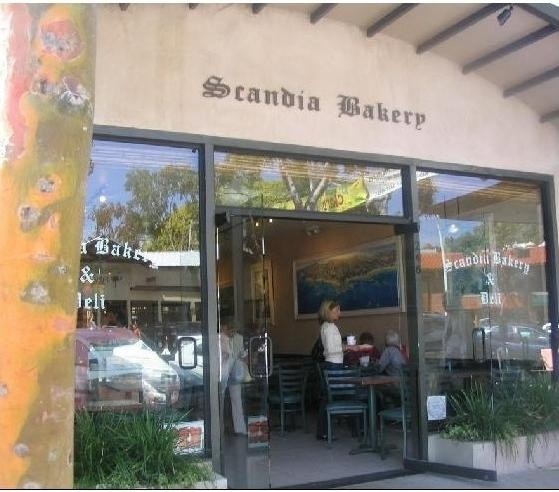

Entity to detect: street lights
[436,222,460,293]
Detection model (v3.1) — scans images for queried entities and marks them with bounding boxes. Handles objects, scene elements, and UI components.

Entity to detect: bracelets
[345,345,347,350]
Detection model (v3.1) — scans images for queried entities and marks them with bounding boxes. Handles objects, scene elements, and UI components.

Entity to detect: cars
[75,323,179,409]
[485,324,551,362]
[138,320,205,409]
[421,313,481,362]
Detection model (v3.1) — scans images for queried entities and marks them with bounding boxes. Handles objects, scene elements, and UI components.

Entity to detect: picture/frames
[293,232,407,320]
[250,258,276,325]
[218,279,239,332]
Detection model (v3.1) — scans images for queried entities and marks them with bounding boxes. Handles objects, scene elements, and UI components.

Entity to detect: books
[342,335,357,345]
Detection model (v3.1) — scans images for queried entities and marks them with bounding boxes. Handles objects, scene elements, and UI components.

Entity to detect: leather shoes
[317,435,336,440]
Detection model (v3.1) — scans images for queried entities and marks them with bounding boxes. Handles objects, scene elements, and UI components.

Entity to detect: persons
[316,298,359,441]
[365,330,410,431]
[423,287,551,370]
[345,332,382,366]
[219,316,252,439]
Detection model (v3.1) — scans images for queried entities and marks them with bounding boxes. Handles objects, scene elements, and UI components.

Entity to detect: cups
[346,335,357,346]
[359,355,370,368]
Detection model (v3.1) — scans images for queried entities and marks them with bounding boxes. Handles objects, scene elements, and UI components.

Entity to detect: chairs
[267,369,308,436]
[85,348,321,419]
[323,369,366,447]
[368,359,528,451]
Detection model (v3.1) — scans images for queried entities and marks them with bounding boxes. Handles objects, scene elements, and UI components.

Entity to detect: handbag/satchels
[230,359,252,383]
[311,336,324,363]
